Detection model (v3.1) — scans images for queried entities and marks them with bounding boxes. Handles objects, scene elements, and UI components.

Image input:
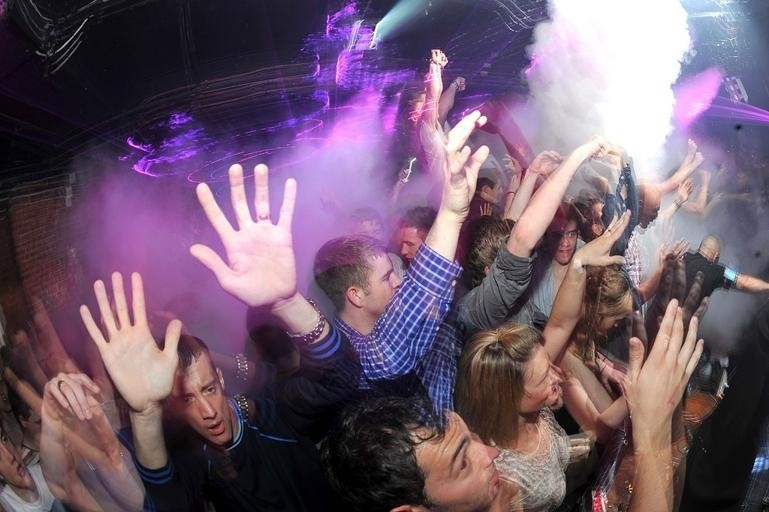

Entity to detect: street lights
[690,348,720,393]
[594,416,693,511]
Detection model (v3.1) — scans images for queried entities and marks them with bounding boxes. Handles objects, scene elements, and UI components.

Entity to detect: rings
[259,216,268,220]
[608,230,612,234]
[58,381,64,388]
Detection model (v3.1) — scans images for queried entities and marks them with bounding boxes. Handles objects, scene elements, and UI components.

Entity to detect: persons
[1,270,299,511]
[312,110,768,512]
[406,50,465,194]
[80,164,359,512]
[306,299,705,511]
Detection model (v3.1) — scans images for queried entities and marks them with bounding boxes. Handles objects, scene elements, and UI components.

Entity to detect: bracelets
[674,200,681,208]
[507,192,515,194]
[233,392,248,420]
[232,353,248,385]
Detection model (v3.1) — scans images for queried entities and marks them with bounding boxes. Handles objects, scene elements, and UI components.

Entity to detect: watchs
[285,298,325,344]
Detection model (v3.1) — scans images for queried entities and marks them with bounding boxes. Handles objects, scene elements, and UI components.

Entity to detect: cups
[551,232,578,239]
